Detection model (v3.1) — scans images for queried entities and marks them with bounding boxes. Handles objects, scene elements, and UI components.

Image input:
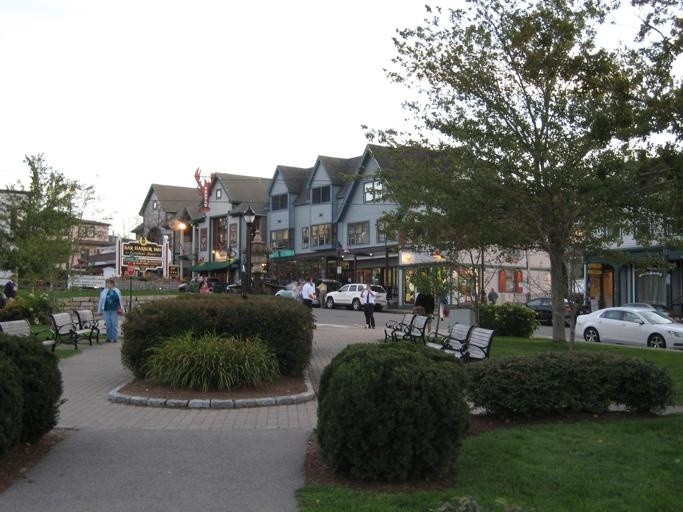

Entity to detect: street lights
[335,241,341,280]
[115,233,121,277]
[172,224,185,281]
[242,205,255,294]
[162,235,169,279]
[227,246,232,284]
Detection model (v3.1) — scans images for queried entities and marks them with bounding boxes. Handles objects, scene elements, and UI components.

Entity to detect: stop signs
[127,262,135,276]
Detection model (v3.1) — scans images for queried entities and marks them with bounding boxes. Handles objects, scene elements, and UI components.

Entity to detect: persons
[488,288,499,304]
[359,283,376,329]
[414,289,448,333]
[3,274,18,298]
[97,278,123,343]
[197,280,211,293]
[292,276,328,311]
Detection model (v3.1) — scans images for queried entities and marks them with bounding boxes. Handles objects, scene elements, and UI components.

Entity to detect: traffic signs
[125,257,139,262]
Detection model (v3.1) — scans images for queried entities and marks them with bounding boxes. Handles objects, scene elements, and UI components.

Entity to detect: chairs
[384,311,495,363]
[0,310,102,352]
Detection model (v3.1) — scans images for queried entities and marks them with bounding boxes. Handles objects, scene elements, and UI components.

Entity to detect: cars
[176,274,340,307]
[523,298,587,328]
[573,307,682,349]
[620,303,672,320]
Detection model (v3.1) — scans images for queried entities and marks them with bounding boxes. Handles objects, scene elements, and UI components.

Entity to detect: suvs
[324,282,388,313]
